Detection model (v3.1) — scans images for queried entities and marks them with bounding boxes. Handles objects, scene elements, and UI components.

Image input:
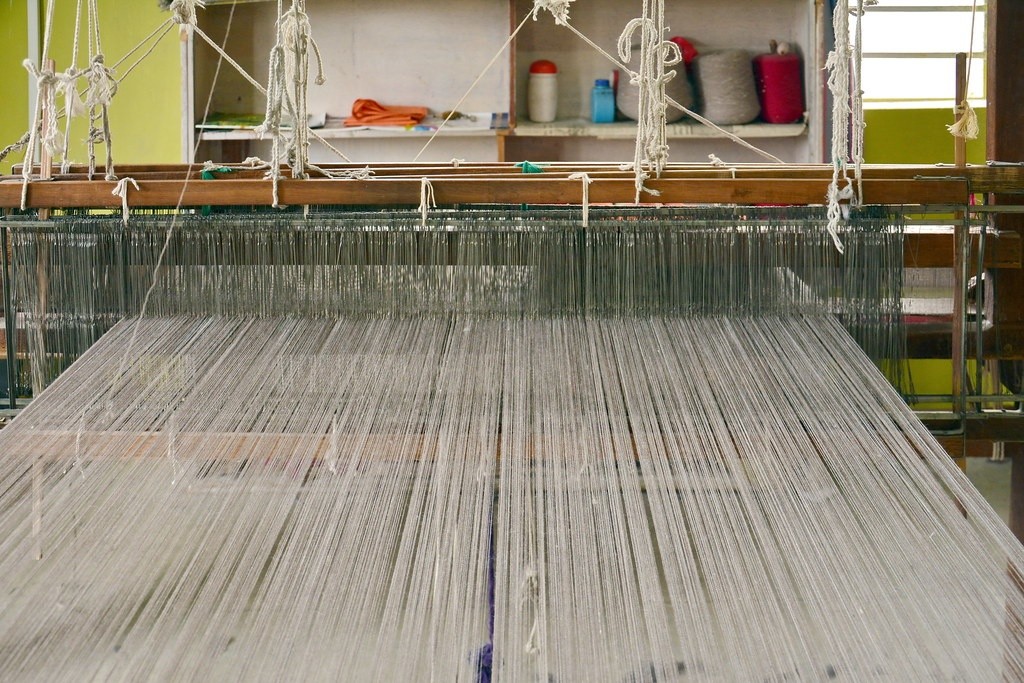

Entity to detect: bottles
[590,79,615,124]
[528,60,559,122]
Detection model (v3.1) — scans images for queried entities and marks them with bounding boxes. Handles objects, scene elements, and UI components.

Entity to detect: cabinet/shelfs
[173,0,836,179]
